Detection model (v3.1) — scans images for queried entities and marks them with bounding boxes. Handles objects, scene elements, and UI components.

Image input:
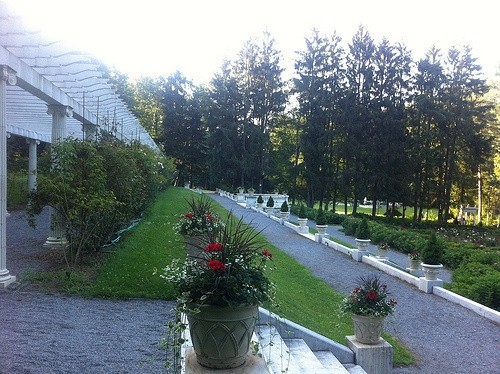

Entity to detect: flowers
[170,191,225,242]
[248,186,255,191]
[333,270,403,348]
[407,249,420,260]
[377,242,390,250]
[153,206,296,374]
[237,186,245,189]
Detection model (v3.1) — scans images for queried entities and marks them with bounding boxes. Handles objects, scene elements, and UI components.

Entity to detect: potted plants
[314,205,328,235]
[354,218,371,251]
[265,195,274,213]
[216,184,238,200]
[296,201,308,227]
[255,195,264,208]
[420,231,444,281]
[280,200,290,218]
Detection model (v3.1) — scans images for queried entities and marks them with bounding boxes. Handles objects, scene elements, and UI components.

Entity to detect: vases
[186,236,210,258]
[184,302,260,369]
[376,249,387,256]
[248,191,254,195]
[410,260,422,270]
[239,189,243,195]
[350,312,387,344]
